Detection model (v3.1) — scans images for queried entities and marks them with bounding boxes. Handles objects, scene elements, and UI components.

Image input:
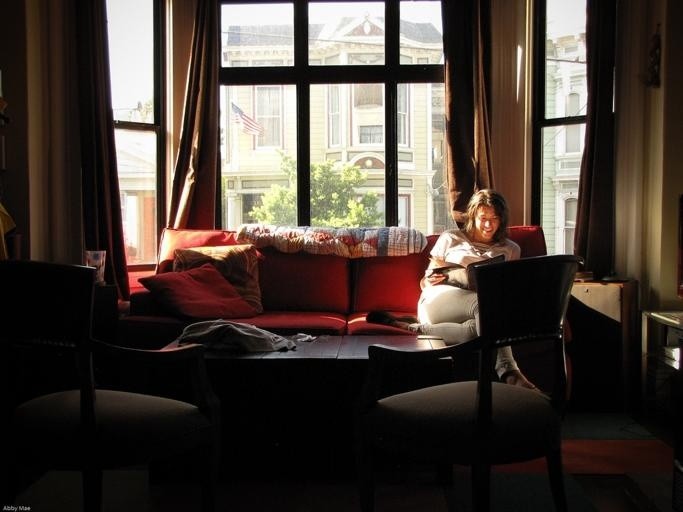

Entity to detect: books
[425,254,505,290]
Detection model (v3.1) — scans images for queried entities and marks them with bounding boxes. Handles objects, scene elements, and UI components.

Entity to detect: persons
[417,189,554,395]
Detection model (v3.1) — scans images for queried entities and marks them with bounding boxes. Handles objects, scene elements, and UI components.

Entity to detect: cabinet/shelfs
[572,278,682,420]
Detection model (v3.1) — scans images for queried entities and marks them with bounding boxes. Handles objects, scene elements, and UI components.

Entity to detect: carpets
[495,439,675,474]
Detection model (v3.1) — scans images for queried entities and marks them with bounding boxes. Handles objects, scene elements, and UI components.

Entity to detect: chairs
[356,256,584,512]
[0,259,215,511]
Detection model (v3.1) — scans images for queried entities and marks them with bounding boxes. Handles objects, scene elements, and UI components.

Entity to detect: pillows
[137,244,264,318]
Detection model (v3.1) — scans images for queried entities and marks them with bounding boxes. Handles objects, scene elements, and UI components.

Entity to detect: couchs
[116,225,573,400]
